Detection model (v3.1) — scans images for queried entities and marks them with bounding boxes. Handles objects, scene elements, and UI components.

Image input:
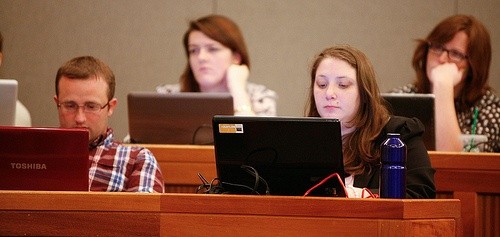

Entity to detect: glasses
[429,44,468,62]
[56,96,113,113]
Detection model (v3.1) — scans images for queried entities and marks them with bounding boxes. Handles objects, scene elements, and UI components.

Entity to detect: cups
[458,134,488,152]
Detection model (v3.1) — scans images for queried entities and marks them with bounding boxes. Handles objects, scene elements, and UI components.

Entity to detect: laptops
[127,92,233,145]
[212,114,345,196]
[380,92,435,150]
[0,126,89,191]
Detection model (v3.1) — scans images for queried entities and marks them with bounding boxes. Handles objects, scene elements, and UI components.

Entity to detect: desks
[0,189,464,237]
[119,143,500,237]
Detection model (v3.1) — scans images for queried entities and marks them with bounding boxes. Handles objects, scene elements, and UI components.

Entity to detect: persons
[0,32,32,127]
[54,55,165,194]
[384,13,500,153]
[124,14,277,145]
[303,44,436,199]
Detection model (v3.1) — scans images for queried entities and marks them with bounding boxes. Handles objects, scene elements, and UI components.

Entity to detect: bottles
[379,133,407,199]
